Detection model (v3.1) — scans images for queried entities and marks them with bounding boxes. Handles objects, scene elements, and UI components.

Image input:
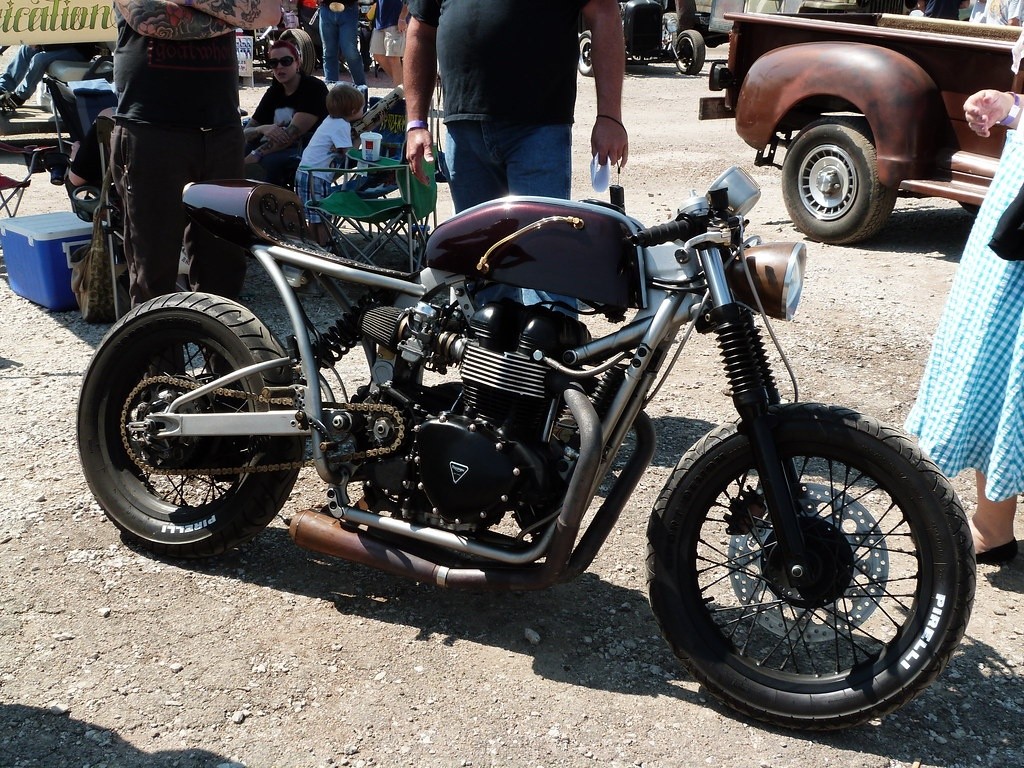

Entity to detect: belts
[121,118,234,139]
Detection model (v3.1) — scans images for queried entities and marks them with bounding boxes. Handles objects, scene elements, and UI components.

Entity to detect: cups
[360,132,382,161]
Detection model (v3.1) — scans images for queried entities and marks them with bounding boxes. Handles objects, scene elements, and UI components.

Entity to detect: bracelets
[251,150,264,159]
[996,92,1020,126]
[407,121,428,132]
[399,19,405,21]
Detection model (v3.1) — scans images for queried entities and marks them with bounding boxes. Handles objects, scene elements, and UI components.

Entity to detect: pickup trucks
[698,11,1024,248]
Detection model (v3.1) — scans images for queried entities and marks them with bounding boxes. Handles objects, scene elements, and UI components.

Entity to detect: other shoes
[973,536,1020,565]
[0,92,26,108]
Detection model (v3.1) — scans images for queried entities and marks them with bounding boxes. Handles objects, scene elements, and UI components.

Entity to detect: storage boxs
[0,211,110,309]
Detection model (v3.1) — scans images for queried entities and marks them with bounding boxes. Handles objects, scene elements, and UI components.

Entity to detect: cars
[578,0,707,78]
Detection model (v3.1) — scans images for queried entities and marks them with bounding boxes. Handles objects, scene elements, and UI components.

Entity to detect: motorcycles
[76,165,978,737]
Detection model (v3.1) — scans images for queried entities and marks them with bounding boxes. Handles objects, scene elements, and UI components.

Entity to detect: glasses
[265,55,297,69]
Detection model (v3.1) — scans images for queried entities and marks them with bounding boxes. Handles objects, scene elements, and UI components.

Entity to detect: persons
[296,85,386,250]
[297,0,412,86]
[68,107,131,292]
[916,0,1024,26]
[403,0,629,320]
[242,40,330,187]
[900,89,1024,566]
[0,42,96,108]
[112,0,280,382]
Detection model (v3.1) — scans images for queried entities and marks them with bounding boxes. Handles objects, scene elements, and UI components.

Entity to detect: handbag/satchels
[66,127,132,326]
[431,148,451,184]
[364,2,377,21]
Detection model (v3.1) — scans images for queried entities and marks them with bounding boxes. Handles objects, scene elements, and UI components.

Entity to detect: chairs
[0,144,55,257]
[290,81,437,297]
